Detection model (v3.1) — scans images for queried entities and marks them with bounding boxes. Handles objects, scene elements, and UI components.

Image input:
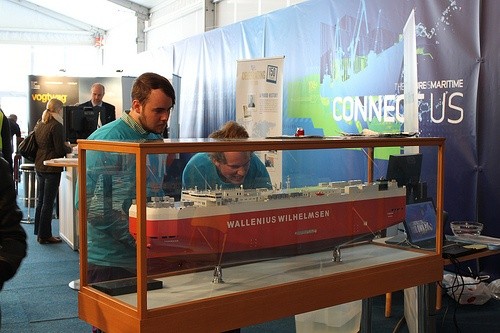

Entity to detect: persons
[184,121,274,192]
[69,83,116,141]
[73,71,176,290]
[14,99,70,244]
[0,109,27,291]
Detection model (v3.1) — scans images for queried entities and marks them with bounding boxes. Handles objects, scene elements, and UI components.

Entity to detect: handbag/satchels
[18,120,40,163]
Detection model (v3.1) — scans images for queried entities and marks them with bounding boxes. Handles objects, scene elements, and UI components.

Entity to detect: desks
[44,158,78,250]
[372,233,499,317]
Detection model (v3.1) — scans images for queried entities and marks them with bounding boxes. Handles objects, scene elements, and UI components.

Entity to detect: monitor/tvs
[385,153,423,192]
[63,105,105,144]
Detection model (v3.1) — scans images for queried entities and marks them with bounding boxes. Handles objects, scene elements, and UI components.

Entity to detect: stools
[20,163,37,224]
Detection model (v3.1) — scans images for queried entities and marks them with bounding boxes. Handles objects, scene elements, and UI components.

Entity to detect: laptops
[403,197,488,257]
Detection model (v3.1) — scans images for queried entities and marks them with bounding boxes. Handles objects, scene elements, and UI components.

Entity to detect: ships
[127,176,407,261]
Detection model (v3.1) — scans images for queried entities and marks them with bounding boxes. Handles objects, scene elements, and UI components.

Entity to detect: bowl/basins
[450,221,483,239]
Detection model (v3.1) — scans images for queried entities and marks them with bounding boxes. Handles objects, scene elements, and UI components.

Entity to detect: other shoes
[39,236,62,244]
[37,234,60,242]
